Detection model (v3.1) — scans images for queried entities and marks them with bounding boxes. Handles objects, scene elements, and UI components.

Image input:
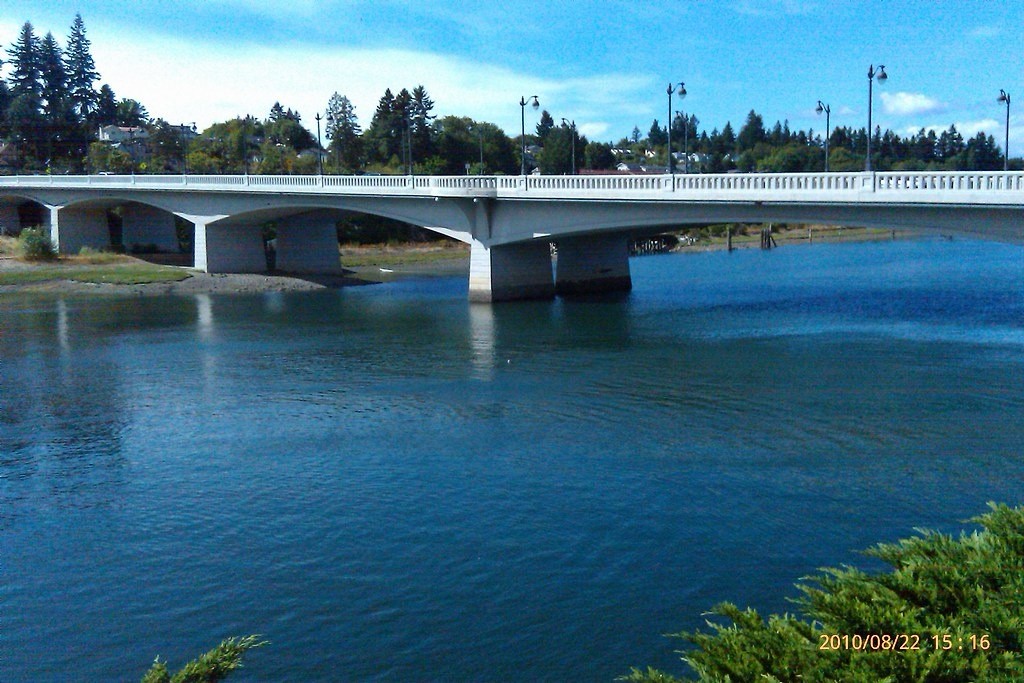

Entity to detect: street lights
[390,129,407,175]
[816,101,830,172]
[519,95,539,175]
[866,65,889,170]
[667,82,687,174]
[674,110,688,174]
[561,117,574,175]
[176,138,186,174]
[315,111,332,176]
[996,89,1010,171]
[468,124,484,176]
[325,133,340,175]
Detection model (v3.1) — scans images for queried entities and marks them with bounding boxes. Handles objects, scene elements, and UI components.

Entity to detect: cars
[362,173,388,185]
[99,172,115,175]
